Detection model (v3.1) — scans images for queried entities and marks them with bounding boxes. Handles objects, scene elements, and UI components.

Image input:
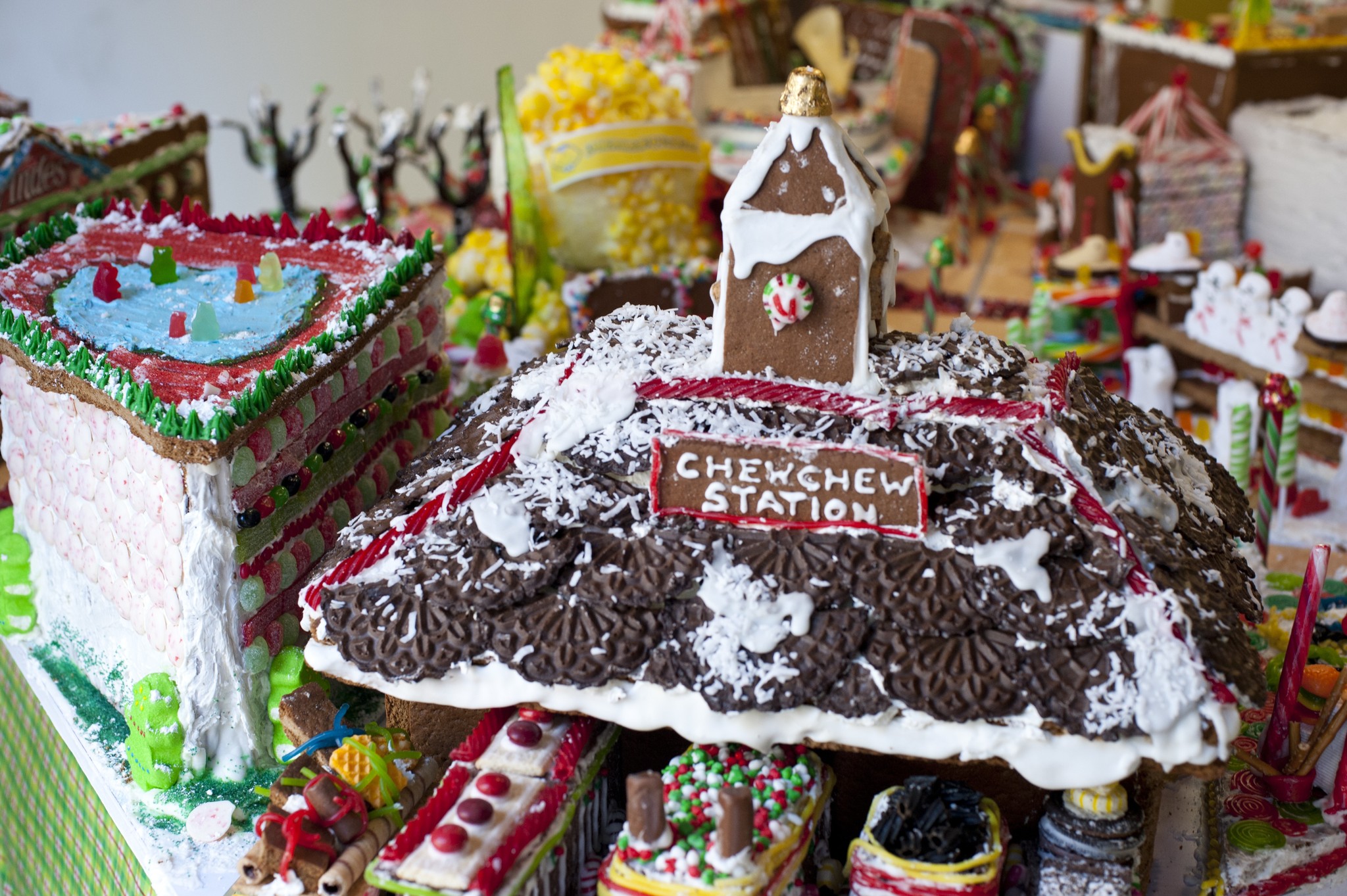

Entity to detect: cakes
[0,1,1347,894]
